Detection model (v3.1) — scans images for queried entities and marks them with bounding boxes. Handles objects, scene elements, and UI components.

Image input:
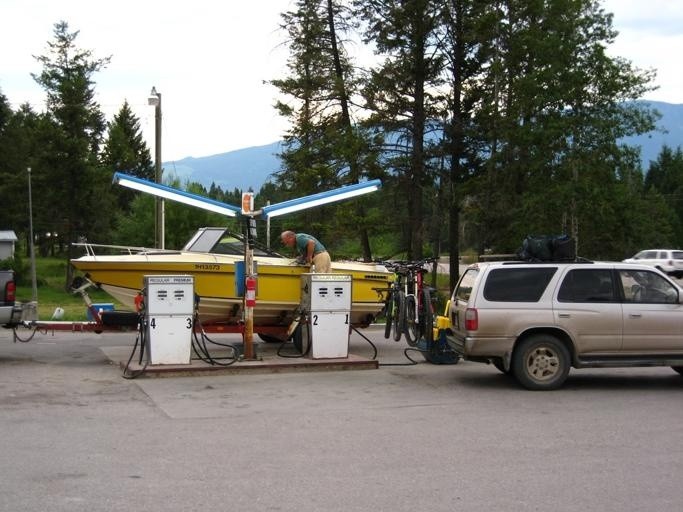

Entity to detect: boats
[68,225,396,342]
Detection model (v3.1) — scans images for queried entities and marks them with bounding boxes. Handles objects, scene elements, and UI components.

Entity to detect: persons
[281,229,333,273]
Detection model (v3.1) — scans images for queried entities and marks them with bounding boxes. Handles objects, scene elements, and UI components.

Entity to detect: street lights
[148,86,165,252]
[25,166,37,319]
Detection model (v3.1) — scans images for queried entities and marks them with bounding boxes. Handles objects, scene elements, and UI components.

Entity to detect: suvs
[445,260,683,388]
[622,249,683,277]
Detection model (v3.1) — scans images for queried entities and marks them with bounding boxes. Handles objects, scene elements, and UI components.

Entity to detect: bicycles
[381,255,440,344]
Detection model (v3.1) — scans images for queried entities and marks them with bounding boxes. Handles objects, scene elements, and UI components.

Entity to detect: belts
[312,252,321,259]
